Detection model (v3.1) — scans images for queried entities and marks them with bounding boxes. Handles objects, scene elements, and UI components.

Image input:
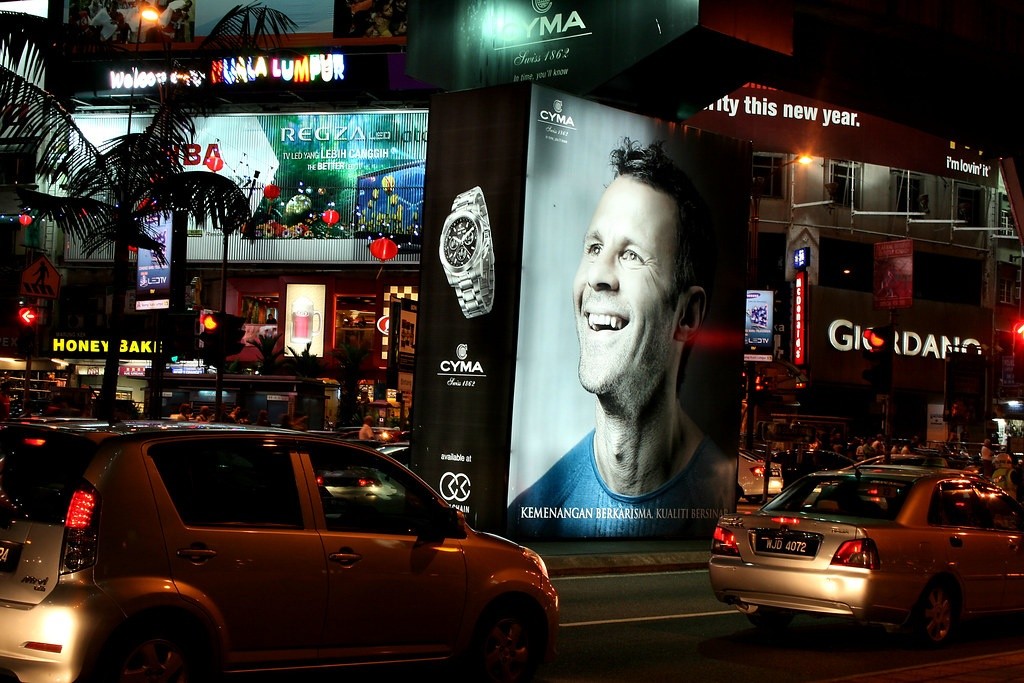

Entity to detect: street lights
[755,156,812,290]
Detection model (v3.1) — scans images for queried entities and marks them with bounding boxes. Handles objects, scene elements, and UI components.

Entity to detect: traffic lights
[202,314,247,366]
[862,322,892,352]
[17,307,39,327]
[1012,317,1024,347]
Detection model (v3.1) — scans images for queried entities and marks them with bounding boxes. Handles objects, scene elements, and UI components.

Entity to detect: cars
[0,419,558,683]
[709,447,1024,646]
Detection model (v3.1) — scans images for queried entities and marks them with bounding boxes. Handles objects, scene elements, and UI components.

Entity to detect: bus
[142,373,325,431]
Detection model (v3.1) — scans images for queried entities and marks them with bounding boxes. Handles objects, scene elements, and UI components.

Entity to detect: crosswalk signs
[21,254,60,300]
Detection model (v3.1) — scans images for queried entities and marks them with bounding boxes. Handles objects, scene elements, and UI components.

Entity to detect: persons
[350,0,408,37]
[245,312,253,323]
[266,314,277,324]
[507,132,737,541]
[359,416,376,440]
[991,452,1024,501]
[342,315,368,347]
[0,381,10,422]
[169,403,309,433]
[980,439,996,478]
[798,428,919,462]
